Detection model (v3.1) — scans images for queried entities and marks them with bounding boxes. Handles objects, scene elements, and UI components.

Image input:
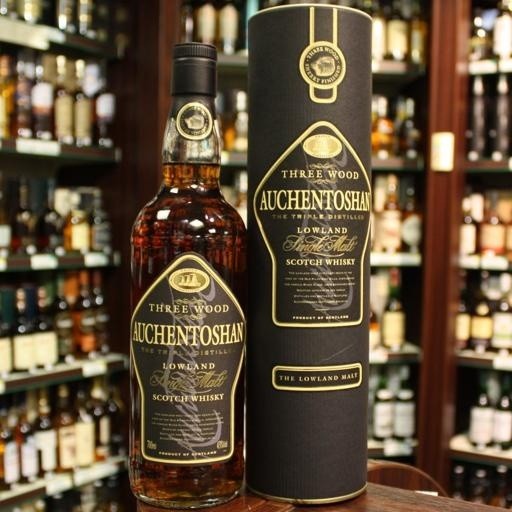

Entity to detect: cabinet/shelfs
[1,0,512,512]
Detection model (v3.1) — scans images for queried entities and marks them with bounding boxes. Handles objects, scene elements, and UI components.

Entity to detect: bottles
[1,1,512,511]
